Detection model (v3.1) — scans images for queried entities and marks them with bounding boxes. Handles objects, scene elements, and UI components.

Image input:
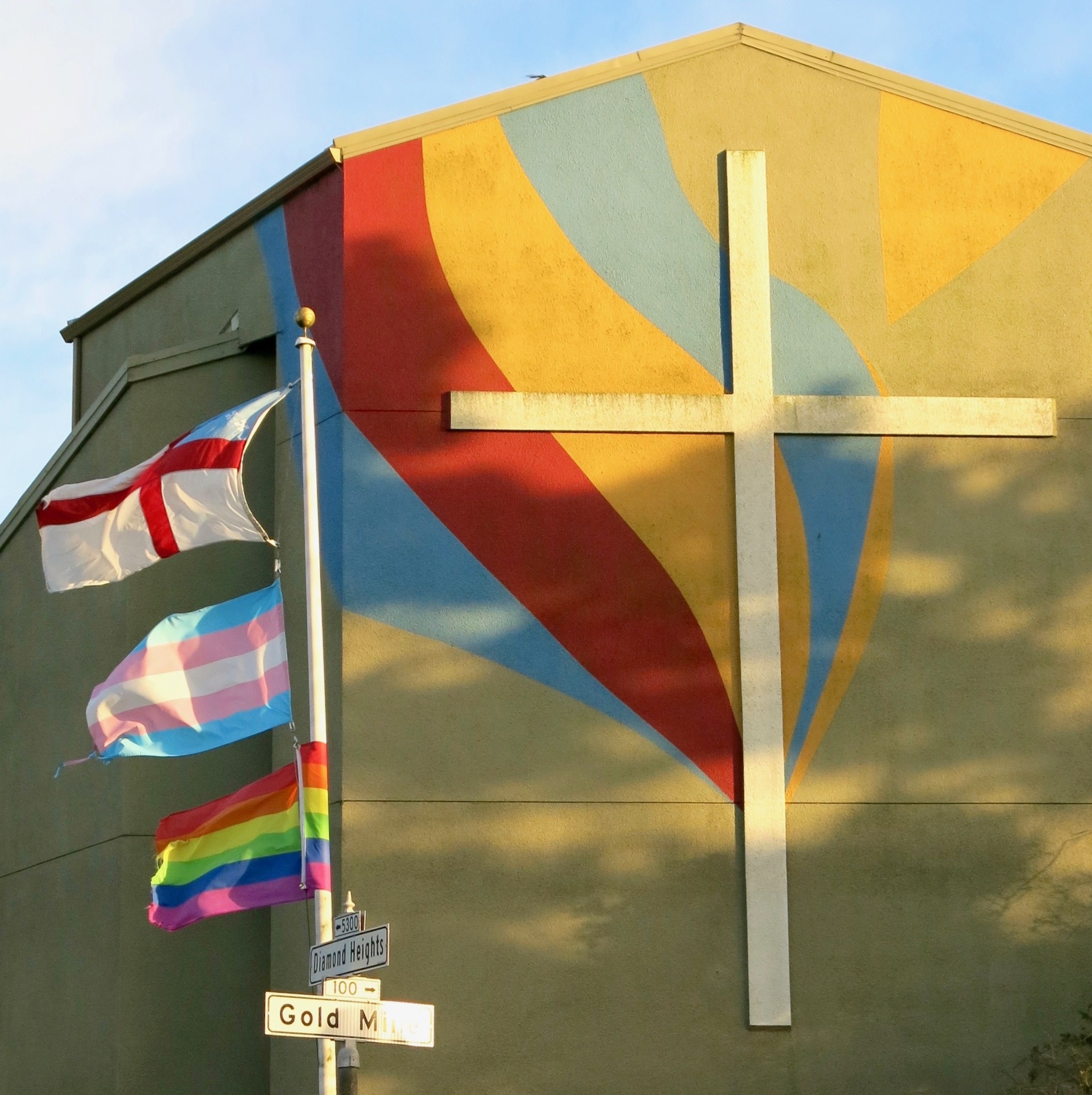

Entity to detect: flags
[35,385,277,597]
[84,575,292,766]
[147,740,331,931]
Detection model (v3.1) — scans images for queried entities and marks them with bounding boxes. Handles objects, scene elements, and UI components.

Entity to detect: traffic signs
[334,910,361,938]
[322,976,381,1000]
